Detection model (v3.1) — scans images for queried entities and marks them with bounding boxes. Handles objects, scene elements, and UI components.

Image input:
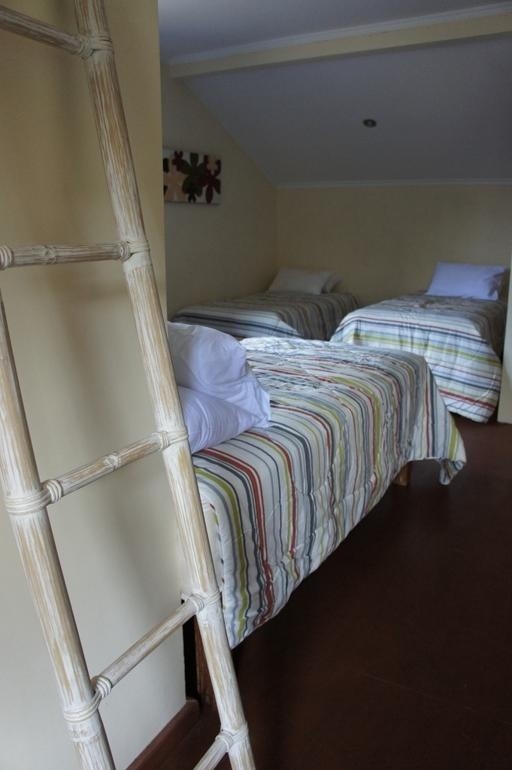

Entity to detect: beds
[169,336,428,700]
[172,286,356,339]
[328,286,507,424]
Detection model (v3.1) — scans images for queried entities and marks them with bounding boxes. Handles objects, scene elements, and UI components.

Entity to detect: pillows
[427,261,508,300]
[268,267,340,293]
[166,318,271,456]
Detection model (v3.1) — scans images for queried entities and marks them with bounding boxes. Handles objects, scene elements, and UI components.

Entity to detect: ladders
[0,1,256,769]
